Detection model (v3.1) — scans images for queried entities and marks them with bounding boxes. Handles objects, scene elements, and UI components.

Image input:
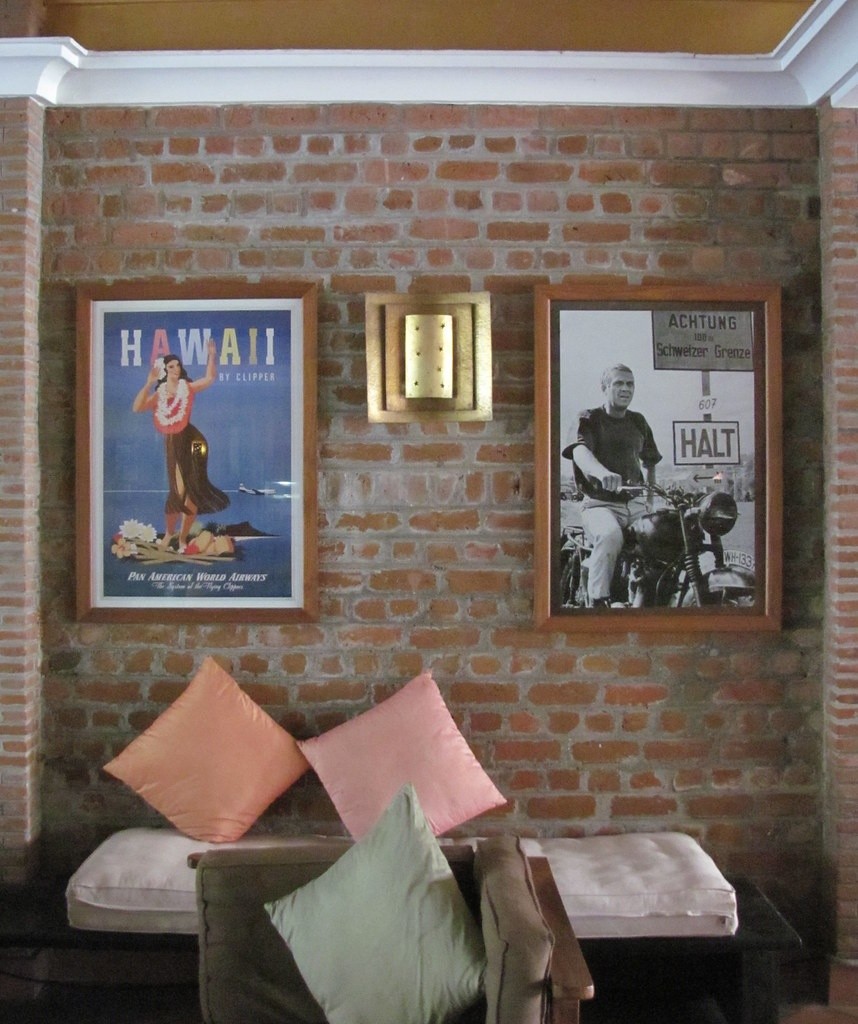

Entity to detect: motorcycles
[560,479,756,607]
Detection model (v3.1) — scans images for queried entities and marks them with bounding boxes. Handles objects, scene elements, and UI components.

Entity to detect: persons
[561,364,662,607]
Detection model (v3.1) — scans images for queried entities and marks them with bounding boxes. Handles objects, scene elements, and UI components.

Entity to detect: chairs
[183,840,596,1024]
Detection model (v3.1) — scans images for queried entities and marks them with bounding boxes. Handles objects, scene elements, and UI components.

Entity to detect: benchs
[0,878,804,1024]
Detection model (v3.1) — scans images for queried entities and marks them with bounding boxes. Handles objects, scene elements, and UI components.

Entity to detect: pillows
[265,783,484,1024]
[102,654,311,842]
[294,667,506,844]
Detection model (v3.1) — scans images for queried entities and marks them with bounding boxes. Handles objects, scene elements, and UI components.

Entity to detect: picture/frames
[70,277,320,624]
[529,281,784,635]
